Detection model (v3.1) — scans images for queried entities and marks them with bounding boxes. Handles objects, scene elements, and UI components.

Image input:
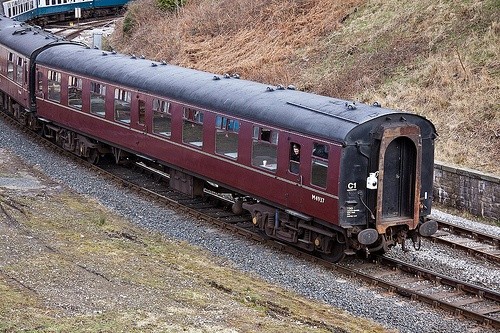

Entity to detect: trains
[0,0,440,265]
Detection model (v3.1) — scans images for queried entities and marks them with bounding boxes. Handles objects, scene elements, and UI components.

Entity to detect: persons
[290,144,301,163]
